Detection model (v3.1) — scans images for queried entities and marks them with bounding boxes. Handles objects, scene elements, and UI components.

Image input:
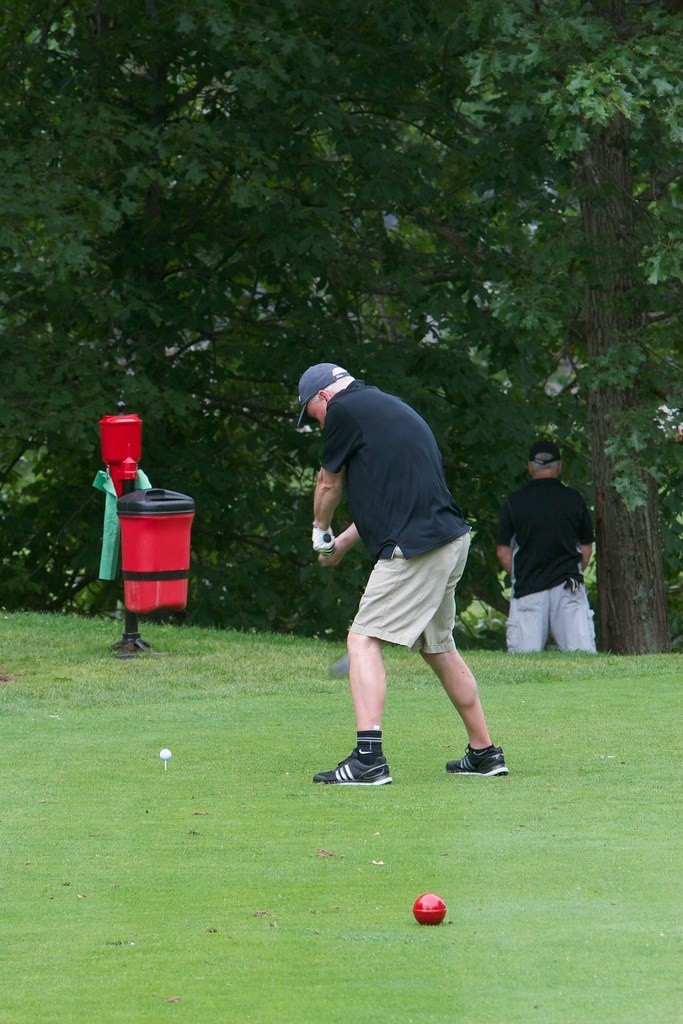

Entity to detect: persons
[297,363,508,786]
[498,442,597,652]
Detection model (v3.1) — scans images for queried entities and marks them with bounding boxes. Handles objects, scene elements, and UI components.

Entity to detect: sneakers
[313,748,392,785]
[446,743,508,776]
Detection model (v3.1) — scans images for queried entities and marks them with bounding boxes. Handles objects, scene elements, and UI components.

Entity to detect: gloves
[312,525,336,556]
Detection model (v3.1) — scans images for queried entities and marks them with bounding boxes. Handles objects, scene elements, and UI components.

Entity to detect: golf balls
[160,749,172,760]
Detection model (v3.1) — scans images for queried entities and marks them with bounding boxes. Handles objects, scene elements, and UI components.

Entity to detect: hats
[529,440,560,465]
[296,363,351,427]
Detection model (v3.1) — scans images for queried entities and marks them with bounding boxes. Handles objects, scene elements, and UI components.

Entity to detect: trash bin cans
[116,488,195,614]
